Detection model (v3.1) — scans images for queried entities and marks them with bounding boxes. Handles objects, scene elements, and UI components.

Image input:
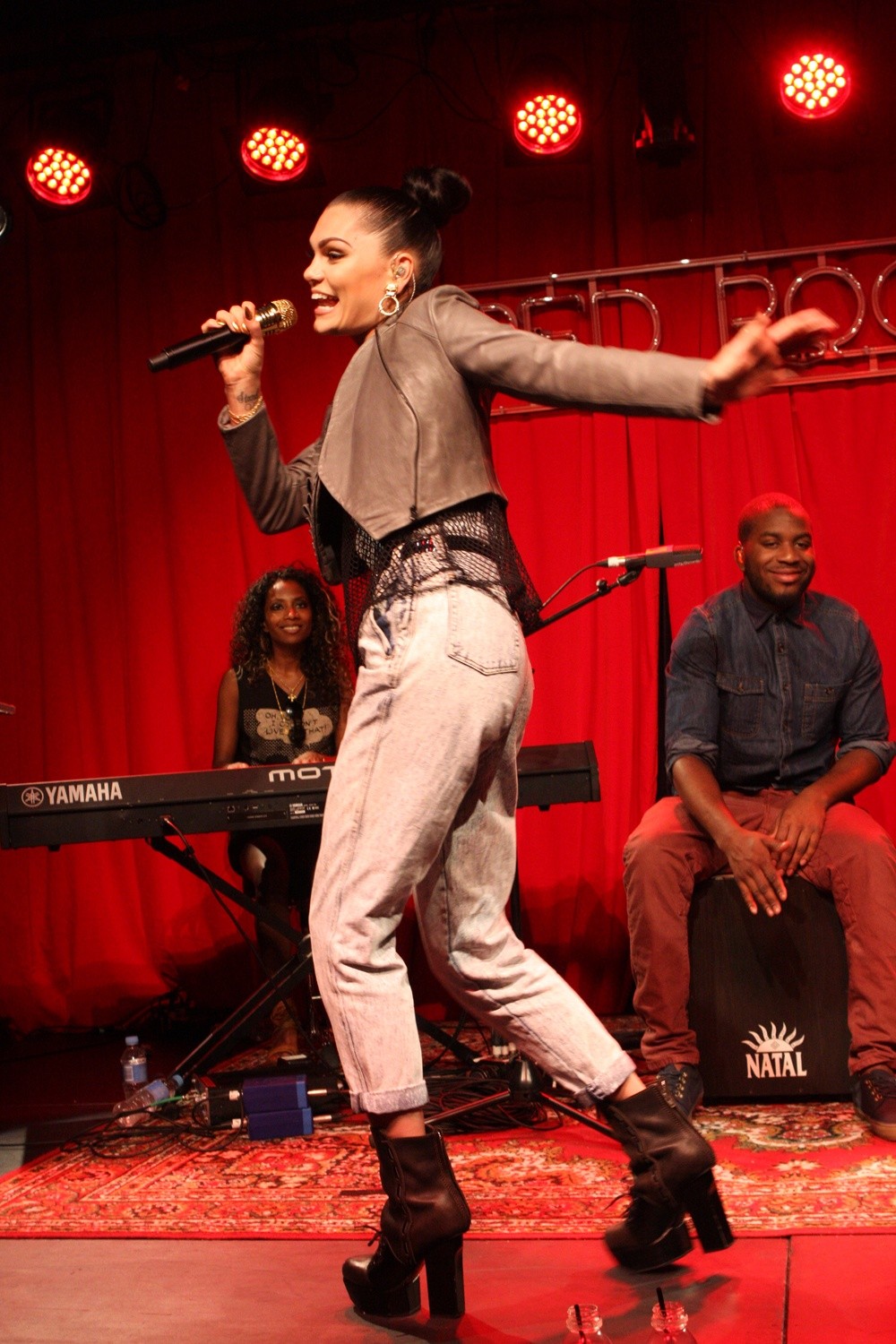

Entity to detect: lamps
[25,31,866,215]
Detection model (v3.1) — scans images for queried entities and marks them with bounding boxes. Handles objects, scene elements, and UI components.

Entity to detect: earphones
[396,263,407,277]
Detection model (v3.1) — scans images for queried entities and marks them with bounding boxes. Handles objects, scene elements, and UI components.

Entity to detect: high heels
[341,1124,471,1318]
[604,1080,733,1274]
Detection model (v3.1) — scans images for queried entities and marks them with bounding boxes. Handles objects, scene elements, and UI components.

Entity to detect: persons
[204,164,842,1316]
[625,492,896,1142]
[212,564,355,1064]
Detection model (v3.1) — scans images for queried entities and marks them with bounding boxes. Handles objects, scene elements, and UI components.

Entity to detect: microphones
[605,542,703,574]
[141,294,301,378]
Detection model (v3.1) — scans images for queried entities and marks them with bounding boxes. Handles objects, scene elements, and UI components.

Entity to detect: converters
[237,1075,308,1113]
[247,1107,314,1139]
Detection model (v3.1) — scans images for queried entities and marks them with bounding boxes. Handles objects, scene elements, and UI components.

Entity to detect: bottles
[177,1087,209,1125]
[120,1037,148,1099]
[112,1075,184,1127]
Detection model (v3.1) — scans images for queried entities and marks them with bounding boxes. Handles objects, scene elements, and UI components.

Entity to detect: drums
[689,874,853,1106]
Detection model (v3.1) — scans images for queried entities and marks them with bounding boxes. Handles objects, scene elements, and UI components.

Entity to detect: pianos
[1,734,606,1085]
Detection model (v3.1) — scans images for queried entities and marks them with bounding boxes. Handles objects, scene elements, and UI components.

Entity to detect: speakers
[688,872,850,1099]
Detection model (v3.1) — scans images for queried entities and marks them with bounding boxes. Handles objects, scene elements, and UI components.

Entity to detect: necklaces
[266,656,311,736]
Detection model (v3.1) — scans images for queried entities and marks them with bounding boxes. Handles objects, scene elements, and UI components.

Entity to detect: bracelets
[226,393,264,424]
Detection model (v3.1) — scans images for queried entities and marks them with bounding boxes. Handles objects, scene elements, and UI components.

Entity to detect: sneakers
[851,1070,896,1141]
[658,1064,706,1122]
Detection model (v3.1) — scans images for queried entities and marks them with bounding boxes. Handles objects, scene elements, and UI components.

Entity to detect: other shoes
[268,1046,298,1065]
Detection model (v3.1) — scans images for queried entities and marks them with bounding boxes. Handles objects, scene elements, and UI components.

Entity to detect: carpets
[1,1065,896,1236]
